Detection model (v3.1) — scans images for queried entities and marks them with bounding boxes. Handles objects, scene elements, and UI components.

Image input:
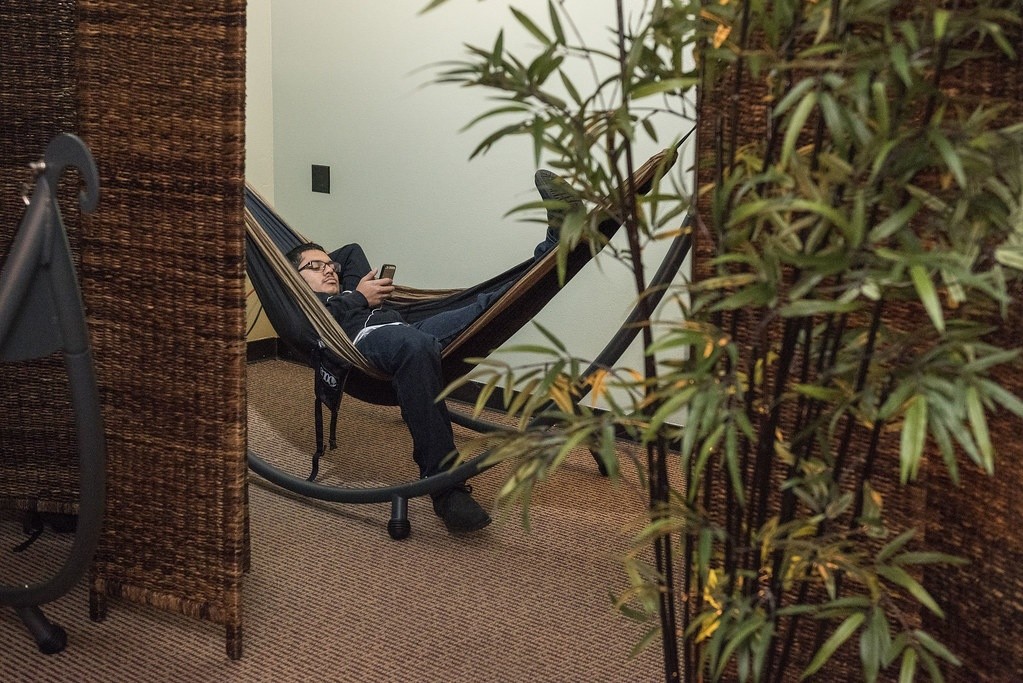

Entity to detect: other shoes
[535,170,586,242]
[433,483,492,533]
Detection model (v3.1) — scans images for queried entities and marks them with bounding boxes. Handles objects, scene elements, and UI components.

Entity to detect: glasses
[297,260,342,272]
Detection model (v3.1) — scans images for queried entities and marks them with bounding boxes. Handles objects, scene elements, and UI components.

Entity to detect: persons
[284,169,588,533]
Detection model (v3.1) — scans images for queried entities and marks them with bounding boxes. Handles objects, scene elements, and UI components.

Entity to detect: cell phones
[380,263,396,285]
